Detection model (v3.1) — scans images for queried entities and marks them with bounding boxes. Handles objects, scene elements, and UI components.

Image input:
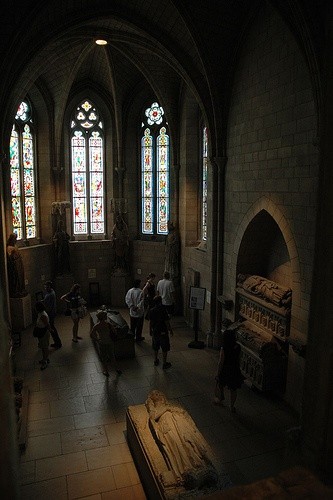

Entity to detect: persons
[41,281,62,347]
[60,284,86,343]
[90,311,119,378]
[157,271,176,319]
[146,389,218,488]
[98,304,128,334]
[110,213,131,270]
[143,272,157,319]
[52,221,71,276]
[33,303,54,370]
[237,272,292,308]
[7,233,29,298]
[163,220,180,280]
[213,330,247,413]
[221,317,278,355]
[149,295,173,370]
[125,279,145,341]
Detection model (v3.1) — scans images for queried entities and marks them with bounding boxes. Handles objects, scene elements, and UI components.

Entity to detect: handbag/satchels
[33,327,47,337]
[108,324,119,342]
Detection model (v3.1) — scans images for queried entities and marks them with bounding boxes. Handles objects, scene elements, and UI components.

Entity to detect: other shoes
[136,337,145,341]
[116,369,123,374]
[72,339,78,343]
[104,373,110,376]
[163,362,171,369]
[51,343,62,347]
[39,359,49,364]
[154,359,159,365]
[77,336,81,339]
[41,364,48,369]
